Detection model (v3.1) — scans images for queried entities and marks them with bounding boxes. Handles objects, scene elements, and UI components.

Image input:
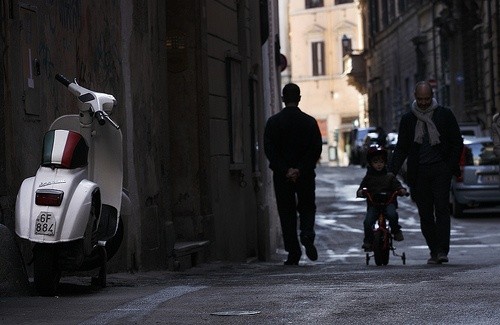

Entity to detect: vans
[348,127,387,165]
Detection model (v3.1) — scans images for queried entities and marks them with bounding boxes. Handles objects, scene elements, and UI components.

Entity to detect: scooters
[11,72,134,297]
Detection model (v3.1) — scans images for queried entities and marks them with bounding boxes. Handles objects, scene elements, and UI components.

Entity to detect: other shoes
[391,229,404,241]
[427,256,442,264]
[436,251,448,262]
[301,239,318,260]
[362,242,374,250]
[283,251,301,265]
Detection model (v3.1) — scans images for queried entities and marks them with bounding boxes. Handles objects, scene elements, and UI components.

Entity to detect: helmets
[365,144,386,164]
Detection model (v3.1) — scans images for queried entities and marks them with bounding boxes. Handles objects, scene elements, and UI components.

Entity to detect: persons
[264,83,323,265]
[390,81,462,264]
[357,151,406,250]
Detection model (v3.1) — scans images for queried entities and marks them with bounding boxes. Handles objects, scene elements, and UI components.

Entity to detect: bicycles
[356,187,410,265]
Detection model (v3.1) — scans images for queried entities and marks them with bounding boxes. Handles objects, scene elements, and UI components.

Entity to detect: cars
[386,132,398,170]
[448,134,500,217]
